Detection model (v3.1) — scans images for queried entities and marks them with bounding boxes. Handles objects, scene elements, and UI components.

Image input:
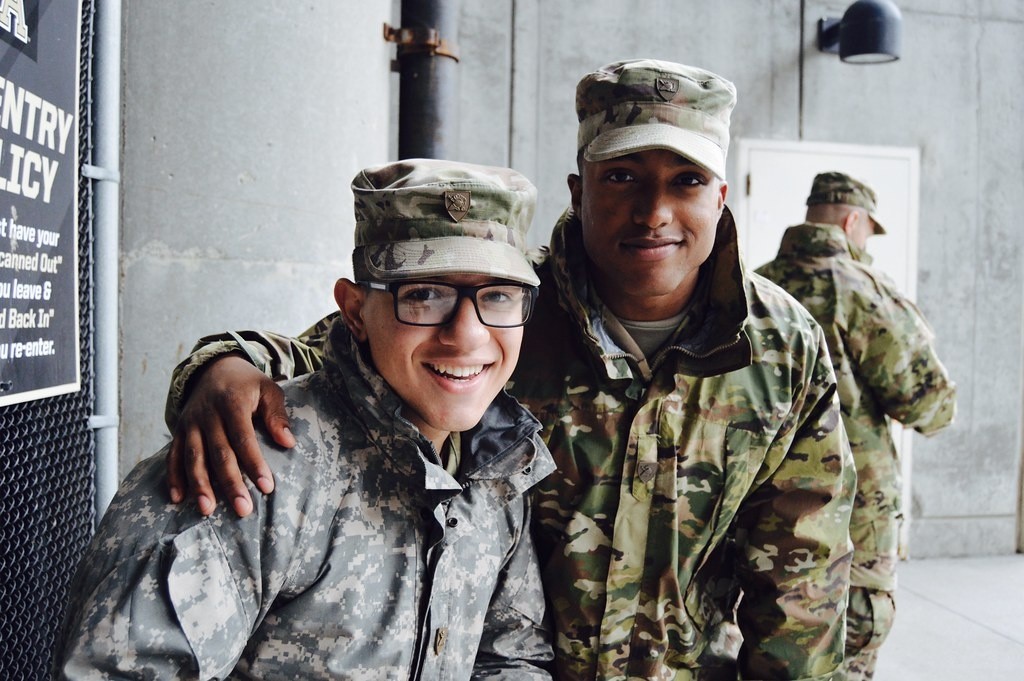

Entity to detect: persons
[52,160,554,680]
[752,168,960,678]
[163,59,860,681]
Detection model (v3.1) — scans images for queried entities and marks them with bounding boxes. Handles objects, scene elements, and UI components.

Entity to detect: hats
[351,158,541,286]
[576,59,737,182]
[806,172,887,234]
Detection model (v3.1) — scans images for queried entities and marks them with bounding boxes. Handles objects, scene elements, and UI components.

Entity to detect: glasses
[356,280,539,328]
